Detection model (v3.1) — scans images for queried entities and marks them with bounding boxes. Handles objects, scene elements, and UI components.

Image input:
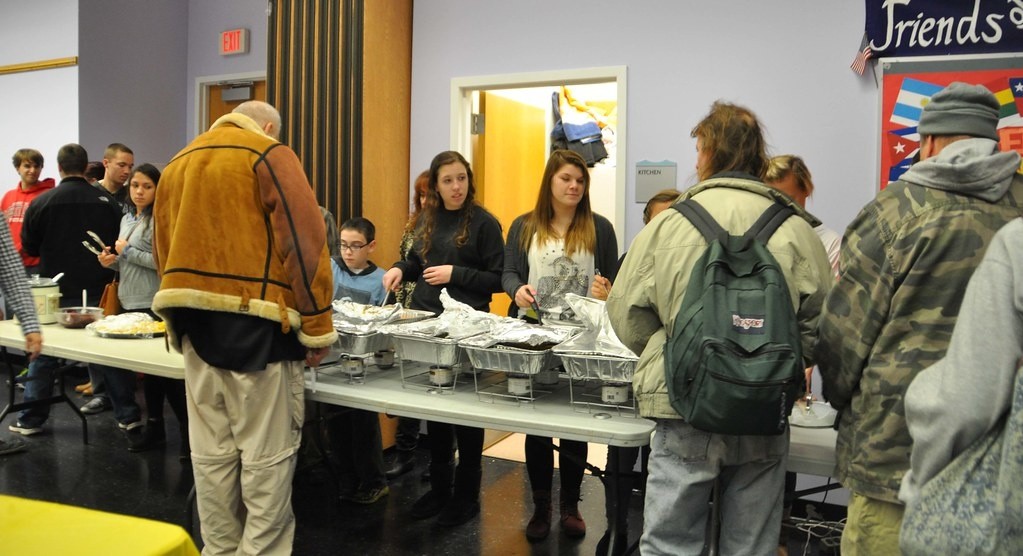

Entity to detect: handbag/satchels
[99,283,122,317]
[900,364,1023,556]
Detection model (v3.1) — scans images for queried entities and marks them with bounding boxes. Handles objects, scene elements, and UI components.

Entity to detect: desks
[0,320,657,448]
[711,400,842,556]
[1,497,203,555]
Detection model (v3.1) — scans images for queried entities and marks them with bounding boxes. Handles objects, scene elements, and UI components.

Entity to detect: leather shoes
[383,452,415,476]
[421,456,434,476]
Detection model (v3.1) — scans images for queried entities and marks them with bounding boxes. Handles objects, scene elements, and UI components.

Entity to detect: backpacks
[661,200,806,436]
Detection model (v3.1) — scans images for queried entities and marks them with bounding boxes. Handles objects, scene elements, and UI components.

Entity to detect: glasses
[338,243,369,251]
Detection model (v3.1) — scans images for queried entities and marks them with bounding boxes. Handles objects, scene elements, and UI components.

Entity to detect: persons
[608,102,838,556]
[0,143,190,459]
[306,151,841,556]
[150,100,338,556]
[816,81,1023,556]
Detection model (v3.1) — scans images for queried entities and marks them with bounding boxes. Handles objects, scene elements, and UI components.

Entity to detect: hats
[917,82,1000,142]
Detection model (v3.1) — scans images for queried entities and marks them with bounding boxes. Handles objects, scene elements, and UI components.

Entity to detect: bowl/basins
[52,307,105,327]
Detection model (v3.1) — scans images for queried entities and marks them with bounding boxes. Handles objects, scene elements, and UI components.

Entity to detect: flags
[850,34,873,75]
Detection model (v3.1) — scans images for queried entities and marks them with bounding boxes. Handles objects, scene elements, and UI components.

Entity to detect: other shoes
[75,382,91,393]
[128,418,167,453]
[80,397,112,413]
[82,387,95,395]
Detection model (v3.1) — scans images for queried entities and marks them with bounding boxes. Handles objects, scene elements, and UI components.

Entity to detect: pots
[13,274,62,325]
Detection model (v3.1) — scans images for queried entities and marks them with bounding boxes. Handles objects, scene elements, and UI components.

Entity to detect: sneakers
[9,421,43,435]
[118,417,144,430]
[352,478,390,504]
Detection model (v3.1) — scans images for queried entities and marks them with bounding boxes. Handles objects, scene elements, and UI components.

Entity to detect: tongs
[82,231,111,257]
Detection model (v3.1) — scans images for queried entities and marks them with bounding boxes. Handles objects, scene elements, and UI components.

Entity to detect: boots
[526,489,552,537]
[411,463,455,519]
[560,488,585,537]
[437,467,482,525]
